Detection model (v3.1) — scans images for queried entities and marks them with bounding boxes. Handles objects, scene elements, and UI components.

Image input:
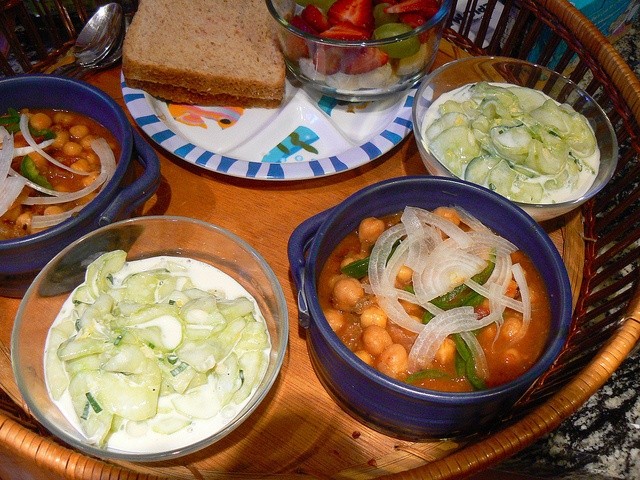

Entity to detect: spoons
[43,3,121,79]
[70,15,133,82]
[61,37,115,77]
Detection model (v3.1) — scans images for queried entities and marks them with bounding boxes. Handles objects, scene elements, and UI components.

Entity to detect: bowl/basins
[10,214,289,463]
[264,0,453,103]
[1,76,163,278]
[288,175,574,437]
[412,55,620,221]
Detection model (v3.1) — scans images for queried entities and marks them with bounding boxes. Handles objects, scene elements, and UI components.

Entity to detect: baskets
[1,1,640,480]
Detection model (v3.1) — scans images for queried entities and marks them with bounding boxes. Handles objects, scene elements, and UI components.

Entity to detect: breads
[119,1,286,109]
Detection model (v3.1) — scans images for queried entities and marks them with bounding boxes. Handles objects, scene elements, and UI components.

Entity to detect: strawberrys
[274,1,442,76]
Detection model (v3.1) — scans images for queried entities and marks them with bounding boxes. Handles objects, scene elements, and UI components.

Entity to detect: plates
[120,73,434,182]
